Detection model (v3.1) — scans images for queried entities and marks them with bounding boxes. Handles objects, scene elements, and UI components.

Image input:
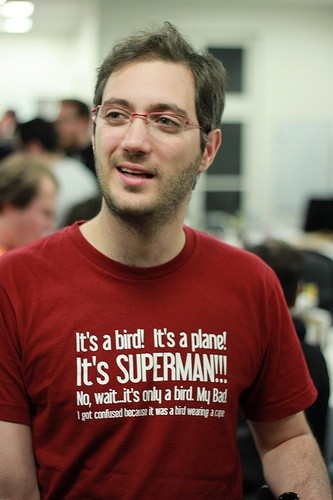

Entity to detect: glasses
[91,105,201,133]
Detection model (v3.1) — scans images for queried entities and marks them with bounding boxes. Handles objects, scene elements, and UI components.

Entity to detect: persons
[0,18,333,500]
